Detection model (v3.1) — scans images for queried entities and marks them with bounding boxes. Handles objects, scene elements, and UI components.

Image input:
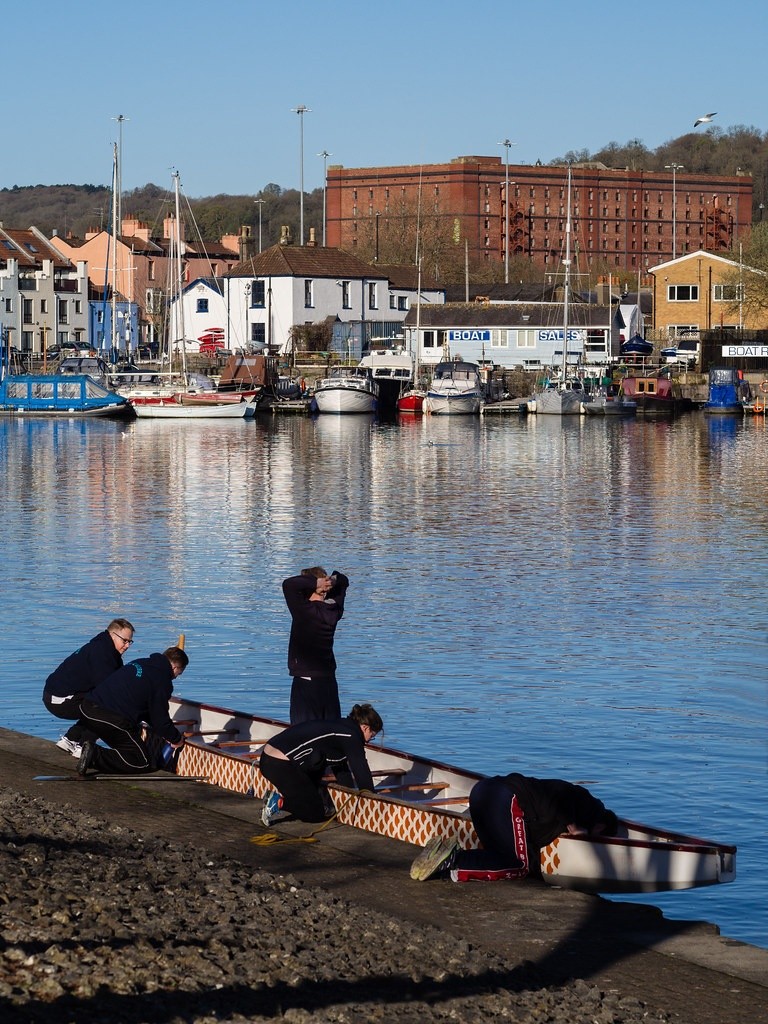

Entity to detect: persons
[260,704,383,826]
[410,773,628,882]
[43,619,134,758]
[76,647,189,776]
[283,567,349,727]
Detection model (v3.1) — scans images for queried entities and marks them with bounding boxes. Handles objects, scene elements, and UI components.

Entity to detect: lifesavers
[760,380,768,393]
[753,403,764,412]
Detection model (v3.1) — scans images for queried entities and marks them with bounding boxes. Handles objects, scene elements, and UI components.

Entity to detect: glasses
[370,730,375,741]
[113,632,134,645]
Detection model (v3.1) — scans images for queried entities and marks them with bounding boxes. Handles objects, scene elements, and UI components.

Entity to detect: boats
[149,697,737,894]
[0,374,130,418]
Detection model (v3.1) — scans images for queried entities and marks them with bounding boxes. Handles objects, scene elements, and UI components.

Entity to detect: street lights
[111,113,132,243]
[253,198,266,254]
[317,150,334,248]
[496,137,518,284]
[290,103,313,247]
[664,160,685,261]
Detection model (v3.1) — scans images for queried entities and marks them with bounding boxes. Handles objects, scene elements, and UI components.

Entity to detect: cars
[1,346,24,365]
[117,363,159,383]
[41,341,97,359]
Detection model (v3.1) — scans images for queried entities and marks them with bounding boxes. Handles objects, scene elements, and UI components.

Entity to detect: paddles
[32,774,208,781]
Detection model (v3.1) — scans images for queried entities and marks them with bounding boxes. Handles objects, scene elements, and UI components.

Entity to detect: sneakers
[259,790,282,827]
[56,737,75,752]
[76,742,97,776]
[418,837,463,882]
[72,743,82,758]
[324,805,335,816]
[410,835,442,880]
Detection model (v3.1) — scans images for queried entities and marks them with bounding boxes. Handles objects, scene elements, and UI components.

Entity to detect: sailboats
[309,157,749,415]
[113,165,263,418]
